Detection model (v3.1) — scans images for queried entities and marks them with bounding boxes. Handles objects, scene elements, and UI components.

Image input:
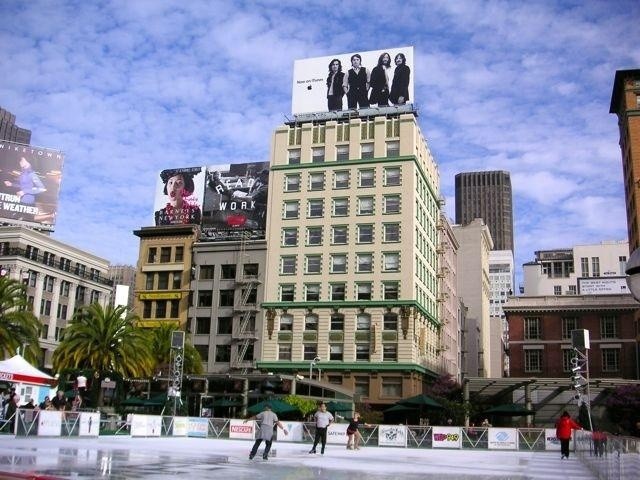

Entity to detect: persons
[370,52,394,108]
[2,153,48,222]
[209,170,236,212]
[417,415,493,448]
[77,371,88,407]
[160,382,180,416]
[389,52,411,105]
[173,382,184,417]
[343,54,372,110]
[592,424,607,458]
[243,403,289,461]
[327,58,348,111]
[309,403,334,454]
[231,169,269,225]
[555,410,584,461]
[297,411,345,442]
[0,387,93,437]
[154,167,201,226]
[334,411,372,451]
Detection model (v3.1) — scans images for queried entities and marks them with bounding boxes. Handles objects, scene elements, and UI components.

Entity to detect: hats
[159,168,201,184]
[24,151,35,165]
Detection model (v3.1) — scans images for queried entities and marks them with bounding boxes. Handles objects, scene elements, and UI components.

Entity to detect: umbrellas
[396,391,446,426]
[0,347,59,409]
[237,394,298,416]
[118,395,149,413]
[145,391,187,406]
[204,398,244,417]
[482,399,537,426]
[382,403,419,424]
[324,400,354,415]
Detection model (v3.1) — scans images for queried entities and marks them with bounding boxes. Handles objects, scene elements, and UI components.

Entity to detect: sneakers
[347,445,360,450]
[309,448,324,454]
[263,454,268,460]
[249,453,254,459]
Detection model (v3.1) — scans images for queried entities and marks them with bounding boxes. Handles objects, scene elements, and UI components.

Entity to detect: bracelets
[282,427,285,431]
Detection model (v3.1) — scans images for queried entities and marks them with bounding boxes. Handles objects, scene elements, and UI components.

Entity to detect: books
[232,190,249,199]
